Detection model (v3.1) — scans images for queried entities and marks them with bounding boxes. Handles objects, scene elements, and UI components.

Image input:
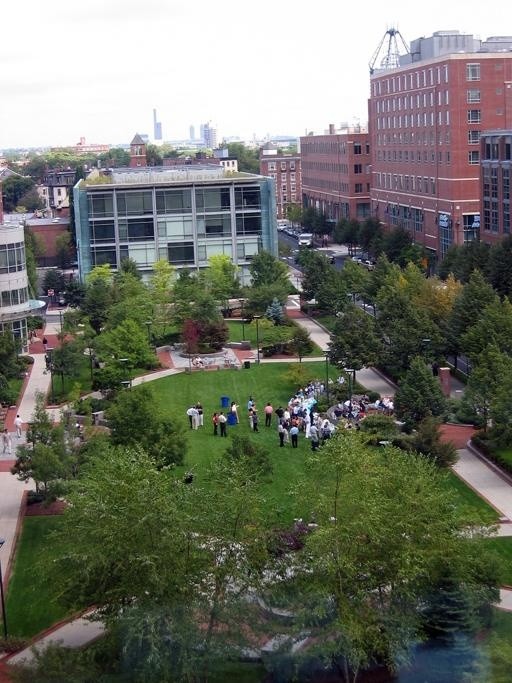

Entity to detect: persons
[195,402,204,426]
[31,332,32,337]
[2,429,11,454]
[213,412,219,435]
[187,407,193,428]
[219,412,227,437]
[43,337,48,350]
[33,331,37,337]
[191,406,199,430]
[192,356,204,366]
[76,420,81,432]
[230,402,239,423]
[15,415,22,438]
[247,376,394,451]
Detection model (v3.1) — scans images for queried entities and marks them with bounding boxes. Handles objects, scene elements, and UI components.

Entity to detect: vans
[299,233,313,247]
[295,249,336,265]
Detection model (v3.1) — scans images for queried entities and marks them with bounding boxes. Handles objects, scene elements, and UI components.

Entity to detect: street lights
[253,316,262,366]
[345,370,354,420]
[46,347,55,402]
[323,350,332,403]
[144,321,152,341]
[238,299,246,341]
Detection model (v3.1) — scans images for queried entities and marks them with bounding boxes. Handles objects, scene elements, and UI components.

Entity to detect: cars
[278,223,302,239]
[352,256,377,271]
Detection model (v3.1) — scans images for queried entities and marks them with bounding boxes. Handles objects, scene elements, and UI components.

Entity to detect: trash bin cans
[221,397,229,408]
[227,412,237,426]
